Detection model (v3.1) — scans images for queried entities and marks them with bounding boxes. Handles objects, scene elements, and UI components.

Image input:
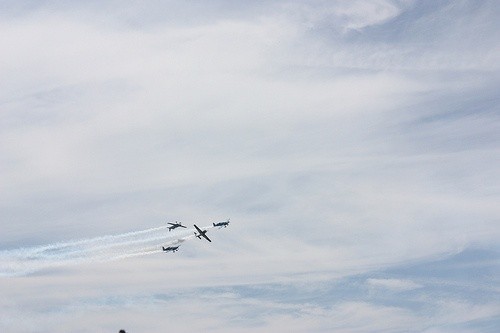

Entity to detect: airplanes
[162,246,179,253]
[213,221,229,229]
[167,221,187,232]
[194,224,211,242]
